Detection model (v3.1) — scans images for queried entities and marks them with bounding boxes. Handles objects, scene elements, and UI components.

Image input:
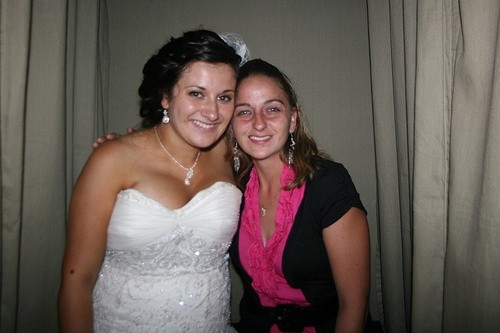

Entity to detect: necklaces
[155,124,200,185]
[259,185,281,216]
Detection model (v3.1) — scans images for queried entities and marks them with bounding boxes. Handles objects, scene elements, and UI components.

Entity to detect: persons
[92,57,386,333]
[58,28,245,333]
